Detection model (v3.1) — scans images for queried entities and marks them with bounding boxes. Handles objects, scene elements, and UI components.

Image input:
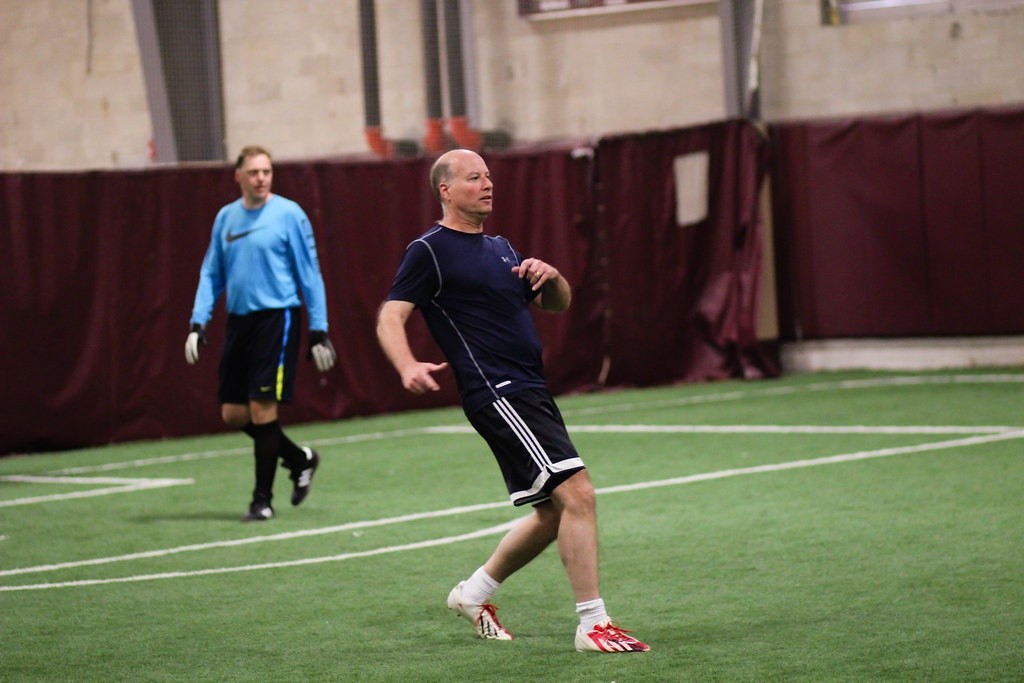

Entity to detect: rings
[536,271,542,276]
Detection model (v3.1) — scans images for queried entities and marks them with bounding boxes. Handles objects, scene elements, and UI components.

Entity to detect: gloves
[185,324,207,364]
[306,331,337,371]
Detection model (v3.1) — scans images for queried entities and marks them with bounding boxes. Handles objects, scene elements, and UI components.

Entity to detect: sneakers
[291,447,319,506]
[241,504,275,522]
[574,621,651,653]
[447,580,513,640]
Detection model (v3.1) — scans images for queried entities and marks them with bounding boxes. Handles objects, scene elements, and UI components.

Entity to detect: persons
[185,144,339,522]
[377,150,651,655]
